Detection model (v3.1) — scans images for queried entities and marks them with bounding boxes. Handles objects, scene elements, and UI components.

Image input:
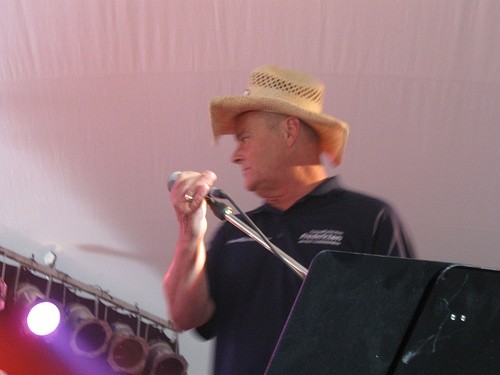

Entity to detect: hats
[211,66,349,168]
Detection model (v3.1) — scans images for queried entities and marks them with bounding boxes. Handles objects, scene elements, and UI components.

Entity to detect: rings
[184,194,193,201]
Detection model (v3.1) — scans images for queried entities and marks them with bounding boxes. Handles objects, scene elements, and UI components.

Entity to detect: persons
[164,60,421,375]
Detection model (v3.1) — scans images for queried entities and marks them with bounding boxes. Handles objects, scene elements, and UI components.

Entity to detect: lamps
[66,303,112,357]
[15,282,69,343]
[107,321,150,375]
[147,339,188,375]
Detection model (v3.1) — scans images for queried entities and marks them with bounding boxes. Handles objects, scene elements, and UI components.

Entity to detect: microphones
[168,171,226,200]
